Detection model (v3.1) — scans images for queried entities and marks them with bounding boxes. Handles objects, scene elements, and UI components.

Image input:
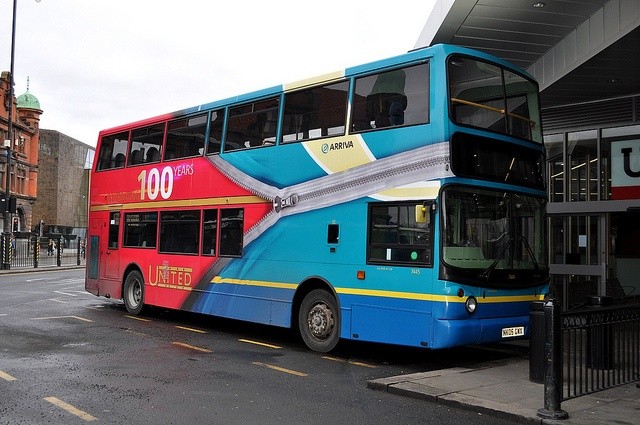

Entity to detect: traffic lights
[14,219,18,230]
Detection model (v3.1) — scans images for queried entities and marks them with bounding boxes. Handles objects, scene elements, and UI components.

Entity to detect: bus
[85,43,549,353]
